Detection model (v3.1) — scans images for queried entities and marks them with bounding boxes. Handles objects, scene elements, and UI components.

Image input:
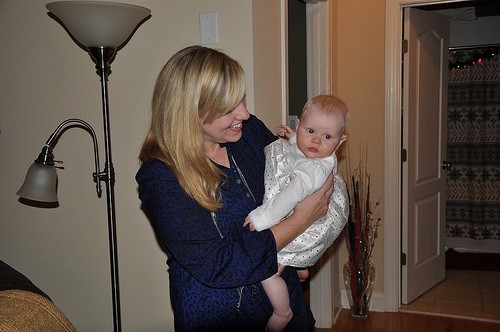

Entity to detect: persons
[243,95,351,332]
[135,45,334,332]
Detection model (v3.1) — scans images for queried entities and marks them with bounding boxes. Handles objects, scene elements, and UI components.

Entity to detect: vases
[343,217,376,321]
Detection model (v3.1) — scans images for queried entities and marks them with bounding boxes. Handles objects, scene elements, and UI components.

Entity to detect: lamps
[16,1,151,332]
